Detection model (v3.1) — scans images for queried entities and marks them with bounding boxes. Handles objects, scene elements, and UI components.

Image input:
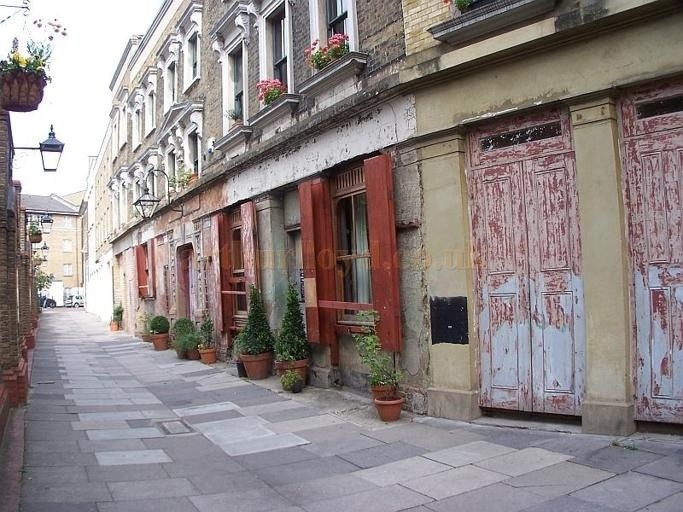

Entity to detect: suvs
[64,294,83,308]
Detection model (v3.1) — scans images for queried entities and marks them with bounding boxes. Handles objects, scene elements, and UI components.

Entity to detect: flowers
[0,36,52,82]
[305,30,348,65]
[442,0,471,11]
[255,78,286,105]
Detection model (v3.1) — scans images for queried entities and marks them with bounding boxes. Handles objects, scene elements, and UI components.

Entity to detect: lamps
[134,167,183,223]
[32,240,48,260]
[13,125,65,171]
[26,210,52,234]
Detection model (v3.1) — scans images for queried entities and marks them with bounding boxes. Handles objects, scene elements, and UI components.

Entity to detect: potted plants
[179,166,198,184]
[109,302,121,330]
[275,278,308,389]
[197,313,217,364]
[279,370,303,394]
[27,224,41,243]
[232,338,246,378]
[353,305,407,421]
[223,108,242,126]
[238,283,272,379]
[135,311,199,360]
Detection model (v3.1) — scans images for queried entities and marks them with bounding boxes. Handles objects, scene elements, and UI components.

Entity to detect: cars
[38,295,57,309]
